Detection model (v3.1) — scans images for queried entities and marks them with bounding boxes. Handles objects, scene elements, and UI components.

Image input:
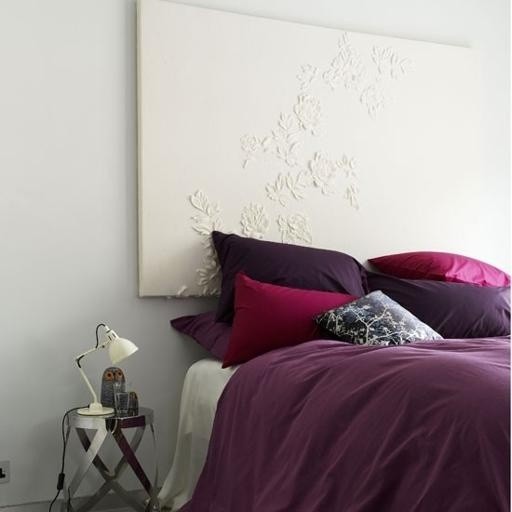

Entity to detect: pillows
[314,290,442,345]
[210,230,367,299]
[171,307,229,362]
[366,251,509,287]
[221,274,359,368]
[364,268,511,338]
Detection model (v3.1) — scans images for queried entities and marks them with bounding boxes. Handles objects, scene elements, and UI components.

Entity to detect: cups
[114,383,130,415]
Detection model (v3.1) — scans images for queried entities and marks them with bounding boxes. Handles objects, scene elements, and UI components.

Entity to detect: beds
[158,337,510,512]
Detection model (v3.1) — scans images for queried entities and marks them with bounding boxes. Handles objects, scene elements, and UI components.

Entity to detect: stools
[59,403,162,512]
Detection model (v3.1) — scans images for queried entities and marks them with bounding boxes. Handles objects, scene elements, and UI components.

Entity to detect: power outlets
[0,460,10,484]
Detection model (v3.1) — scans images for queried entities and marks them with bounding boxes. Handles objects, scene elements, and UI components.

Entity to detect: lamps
[73,323,138,415]
